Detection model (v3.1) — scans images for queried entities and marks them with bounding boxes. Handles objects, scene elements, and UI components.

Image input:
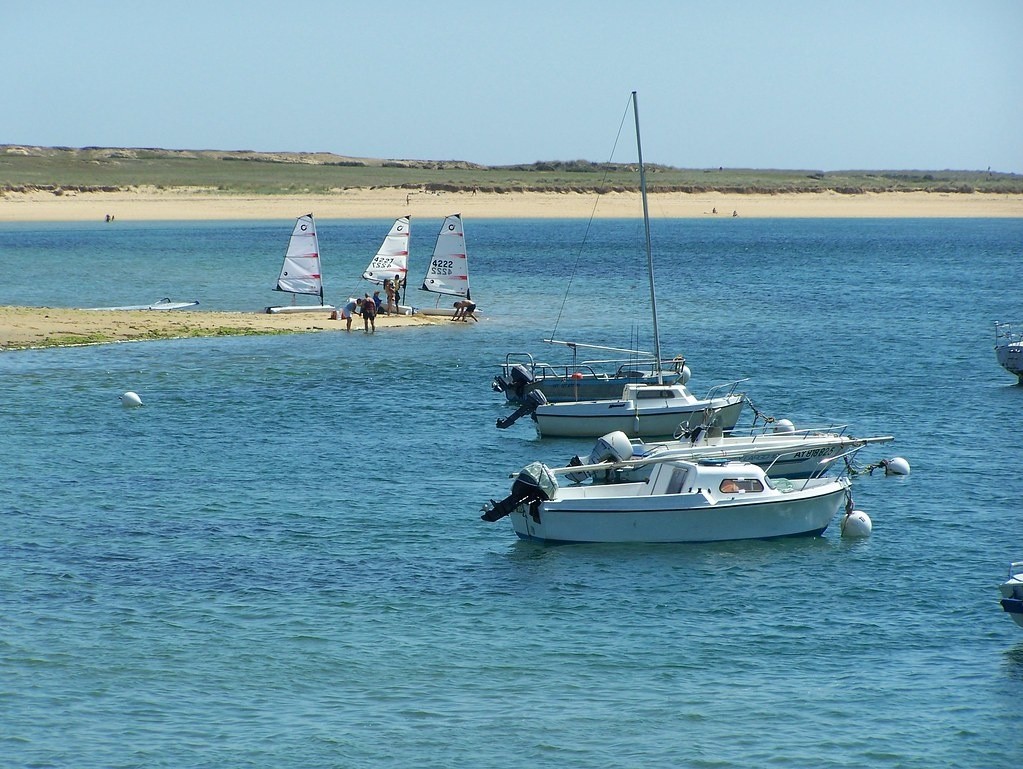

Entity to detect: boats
[999,562,1023,625]
[992,320,1023,379]
[479,91,910,543]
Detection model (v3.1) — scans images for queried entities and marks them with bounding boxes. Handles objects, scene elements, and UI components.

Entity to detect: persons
[713,208,717,213]
[105,214,115,223]
[452,300,478,322]
[343,291,382,334]
[384,274,405,316]
[732,210,739,216]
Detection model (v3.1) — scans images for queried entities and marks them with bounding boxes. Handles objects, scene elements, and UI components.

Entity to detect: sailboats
[265,213,483,317]
